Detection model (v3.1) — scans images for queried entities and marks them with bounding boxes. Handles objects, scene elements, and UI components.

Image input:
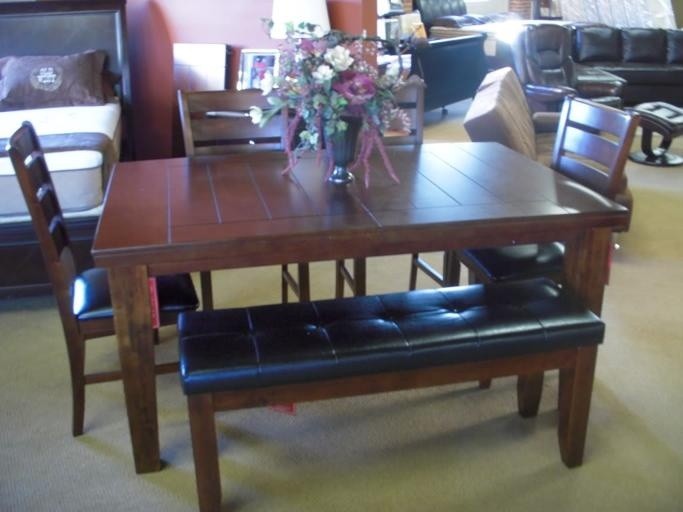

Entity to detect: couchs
[511,21,683,108]
[461,67,635,234]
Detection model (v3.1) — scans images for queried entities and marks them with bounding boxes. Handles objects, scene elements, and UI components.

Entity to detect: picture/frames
[234,48,280,91]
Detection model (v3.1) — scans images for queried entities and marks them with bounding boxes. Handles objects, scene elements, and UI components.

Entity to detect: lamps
[267,0,333,64]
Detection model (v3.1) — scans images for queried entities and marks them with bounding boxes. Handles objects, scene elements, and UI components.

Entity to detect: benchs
[170,272,608,511]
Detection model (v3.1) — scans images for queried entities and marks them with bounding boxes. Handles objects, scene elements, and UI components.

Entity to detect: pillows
[0,46,111,117]
[102,69,124,104]
[433,10,520,30]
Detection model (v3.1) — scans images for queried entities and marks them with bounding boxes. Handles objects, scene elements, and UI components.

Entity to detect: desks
[623,102,683,168]
[89,137,634,476]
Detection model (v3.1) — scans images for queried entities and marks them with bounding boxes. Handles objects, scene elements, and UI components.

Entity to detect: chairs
[173,86,313,312]
[3,120,211,442]
[447,88,641,393]
[399,31,491,117]
[332,76,465,300]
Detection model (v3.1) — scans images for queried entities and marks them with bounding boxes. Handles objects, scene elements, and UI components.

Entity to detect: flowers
[247,16,432,189]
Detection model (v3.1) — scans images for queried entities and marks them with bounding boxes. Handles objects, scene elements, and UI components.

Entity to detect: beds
[412,1,571,59]
[0,0,135,301]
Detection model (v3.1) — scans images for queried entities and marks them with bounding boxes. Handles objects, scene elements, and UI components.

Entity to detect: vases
[319,115,365,184]
